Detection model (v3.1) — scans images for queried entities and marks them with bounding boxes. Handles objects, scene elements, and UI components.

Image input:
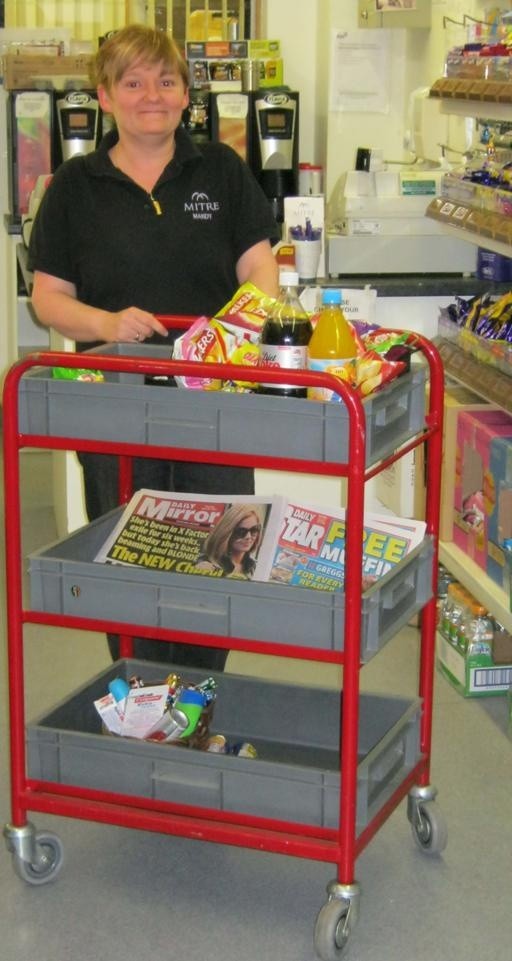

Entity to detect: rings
[135,332,140,340]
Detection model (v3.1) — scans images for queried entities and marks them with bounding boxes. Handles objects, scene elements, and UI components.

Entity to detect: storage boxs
[22,653,426,844]
[25,502,436,670]
[14,337,423,470]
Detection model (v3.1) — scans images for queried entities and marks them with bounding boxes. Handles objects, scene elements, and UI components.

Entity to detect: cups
[290,236,323,279]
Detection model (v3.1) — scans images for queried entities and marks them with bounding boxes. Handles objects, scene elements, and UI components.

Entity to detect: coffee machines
[249,84,299,225]
[54,87,102,171]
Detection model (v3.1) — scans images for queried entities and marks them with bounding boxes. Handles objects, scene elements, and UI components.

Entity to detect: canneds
[141,709,189,744]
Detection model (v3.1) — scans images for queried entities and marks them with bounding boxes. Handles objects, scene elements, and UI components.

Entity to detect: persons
[25,25,282,672]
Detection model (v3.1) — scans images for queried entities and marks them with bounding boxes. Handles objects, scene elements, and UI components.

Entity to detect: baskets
[101,679,215,751]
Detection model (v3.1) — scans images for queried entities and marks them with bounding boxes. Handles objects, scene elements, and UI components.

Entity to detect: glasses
[232,524,262,538]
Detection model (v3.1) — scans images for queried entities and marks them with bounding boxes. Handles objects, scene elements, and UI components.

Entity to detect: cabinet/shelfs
[423,76,512,737]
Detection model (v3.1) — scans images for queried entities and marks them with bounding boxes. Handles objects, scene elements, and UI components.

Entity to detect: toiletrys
[174,685,205,737]
[147,709,189,743]
[108,679,129,711]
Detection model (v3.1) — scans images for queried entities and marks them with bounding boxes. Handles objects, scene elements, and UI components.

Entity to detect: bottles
[107,677,132,715]
[437,563,494,667]
[258,270,316,399]
[311,166,322,195]
[305,286,359,401]
[297,162,311,196]
